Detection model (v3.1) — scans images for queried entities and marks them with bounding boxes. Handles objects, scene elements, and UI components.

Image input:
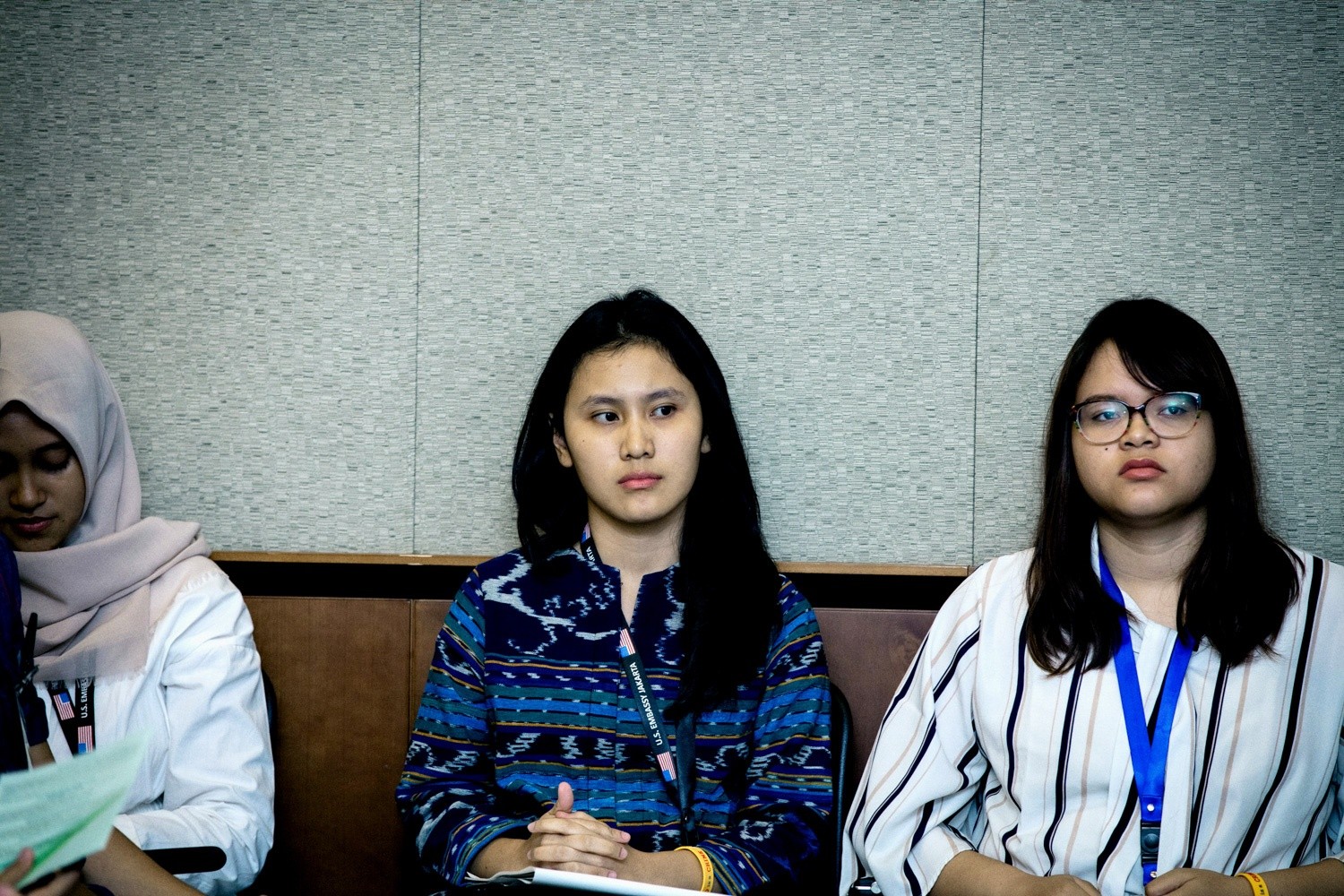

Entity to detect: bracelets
[674,845,714,893]
[1235,872,1269,896]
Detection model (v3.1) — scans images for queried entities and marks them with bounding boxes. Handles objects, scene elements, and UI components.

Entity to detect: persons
[0,526,209,896]
[0,310,276,896]
[398,291,841,896]
[848,297,1344,896]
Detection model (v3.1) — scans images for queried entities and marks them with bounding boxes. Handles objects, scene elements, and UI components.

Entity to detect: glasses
[1069,390,1202,446]
